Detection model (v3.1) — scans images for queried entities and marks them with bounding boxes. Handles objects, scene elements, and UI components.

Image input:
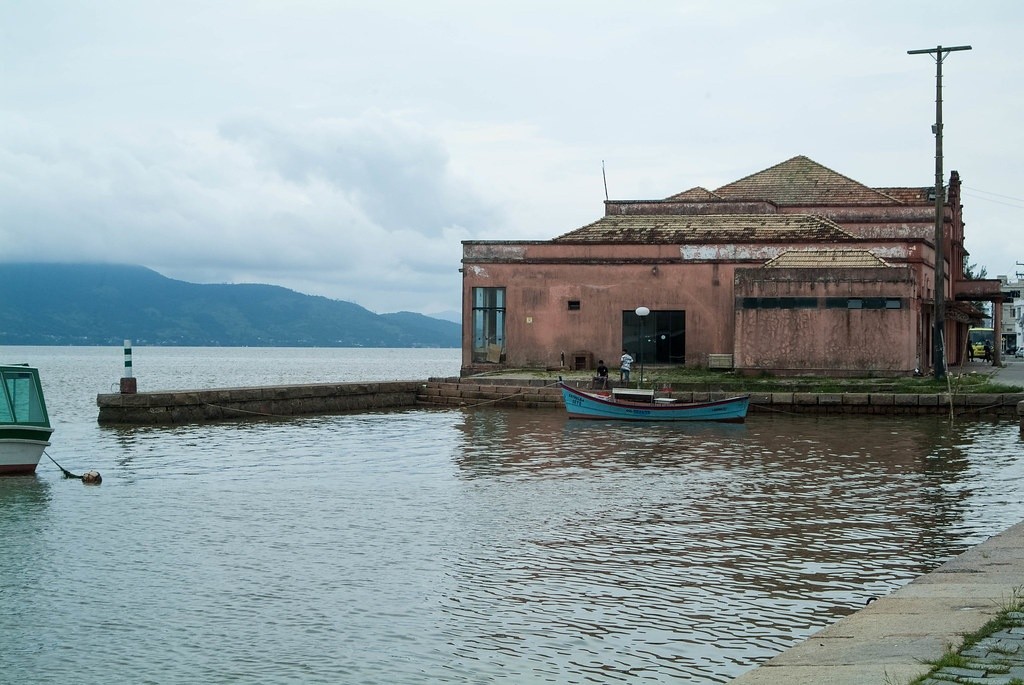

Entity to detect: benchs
[654,398,678,406]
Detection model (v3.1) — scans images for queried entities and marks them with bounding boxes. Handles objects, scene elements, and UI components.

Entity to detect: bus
[967,327,995,358]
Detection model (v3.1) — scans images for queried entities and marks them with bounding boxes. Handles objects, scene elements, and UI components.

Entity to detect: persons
[968,335,974,362]
[619,349,633,386]
[589,360,608,390]
[982,341,993,363]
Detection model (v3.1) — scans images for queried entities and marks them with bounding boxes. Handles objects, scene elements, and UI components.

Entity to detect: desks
[612,388,655,407]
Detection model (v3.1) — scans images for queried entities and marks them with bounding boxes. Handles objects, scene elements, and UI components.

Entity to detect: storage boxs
[708,353,734,368]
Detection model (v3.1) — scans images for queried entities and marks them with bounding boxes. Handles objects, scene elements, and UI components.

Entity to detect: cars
[1006,345,1016,354]
[1015,346,1024,358]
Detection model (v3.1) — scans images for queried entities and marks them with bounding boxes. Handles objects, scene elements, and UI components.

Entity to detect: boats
[0,362,54,476]
[559,376,751,424]
